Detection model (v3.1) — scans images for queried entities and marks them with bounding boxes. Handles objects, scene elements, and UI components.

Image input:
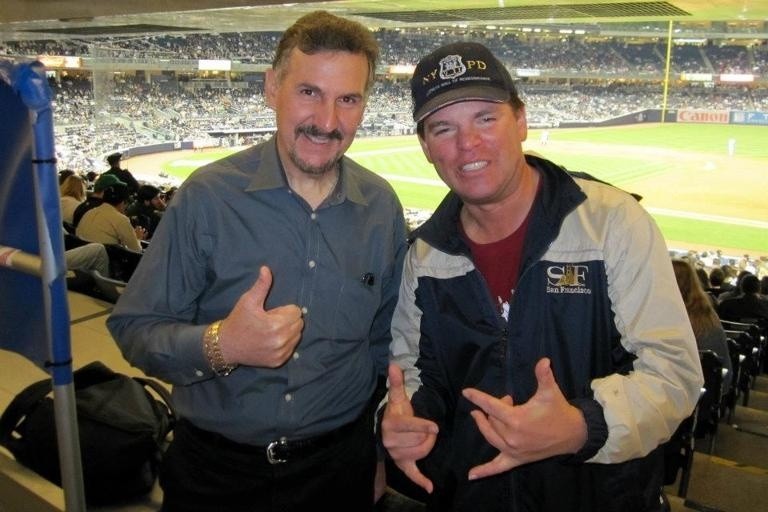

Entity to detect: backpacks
[0,359,180,508]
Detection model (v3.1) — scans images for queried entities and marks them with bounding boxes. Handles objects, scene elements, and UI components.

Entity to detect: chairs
[663,319,765,494]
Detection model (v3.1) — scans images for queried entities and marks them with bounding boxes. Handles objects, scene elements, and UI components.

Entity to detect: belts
[181,407,371,465]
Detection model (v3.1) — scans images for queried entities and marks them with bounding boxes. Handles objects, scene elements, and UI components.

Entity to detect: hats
[107,153,123,162]
[409,42,518,124]
[92,174,128,191]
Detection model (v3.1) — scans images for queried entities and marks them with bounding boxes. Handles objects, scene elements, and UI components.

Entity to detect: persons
[671,246,768,431]
[484,31,767,153]
[0,33,285,155]
[399,202,434,229]
[112,8,410,511]
[373,40,707,511]
[53,154,180,307]
[354,28,488,139]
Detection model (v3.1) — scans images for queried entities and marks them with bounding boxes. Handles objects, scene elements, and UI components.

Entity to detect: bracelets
[205,321,237,377]
